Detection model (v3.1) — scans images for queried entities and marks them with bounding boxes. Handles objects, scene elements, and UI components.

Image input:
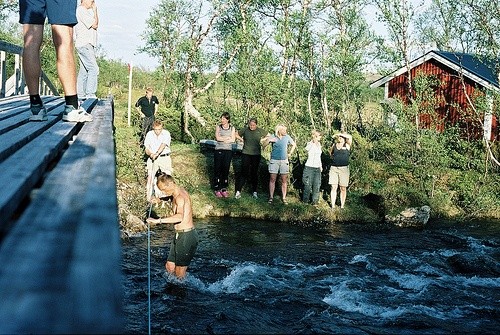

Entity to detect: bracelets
[156,151,161,155]
[160,218,161,224]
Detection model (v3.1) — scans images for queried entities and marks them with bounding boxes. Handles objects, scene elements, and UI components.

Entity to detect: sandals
[268,197,274,204]
[282,198,288,205]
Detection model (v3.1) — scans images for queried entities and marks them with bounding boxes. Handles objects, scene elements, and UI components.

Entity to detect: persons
[144,120,171,204]
[328,133,352,209]
[263,124,296,205]
[19,0,93,122]
[146,169,198,281]
[134,88,159,148]
[73,0,101,101]
[235,118,270,199]
[301,129,323,206]
[213,112,235,197]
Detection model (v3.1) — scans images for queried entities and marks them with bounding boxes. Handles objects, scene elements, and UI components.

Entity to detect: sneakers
[29,107,48,121]
[61,105,93,122]
